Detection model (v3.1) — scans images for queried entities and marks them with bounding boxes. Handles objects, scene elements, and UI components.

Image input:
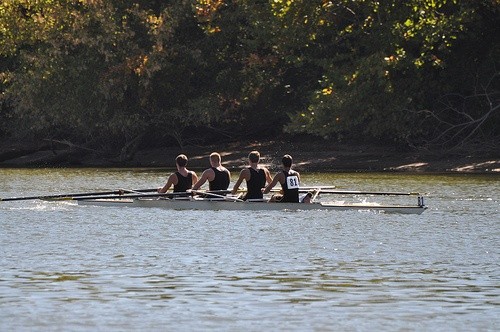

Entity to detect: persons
[262,155,300,203]
[232,151,273,201]
[191,152,231,198]
[158,154,201,199]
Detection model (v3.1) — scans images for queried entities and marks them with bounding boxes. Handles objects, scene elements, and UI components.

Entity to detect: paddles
[0,185,337,202]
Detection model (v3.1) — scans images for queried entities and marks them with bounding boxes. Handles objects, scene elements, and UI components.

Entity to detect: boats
[65,188,429,214]
